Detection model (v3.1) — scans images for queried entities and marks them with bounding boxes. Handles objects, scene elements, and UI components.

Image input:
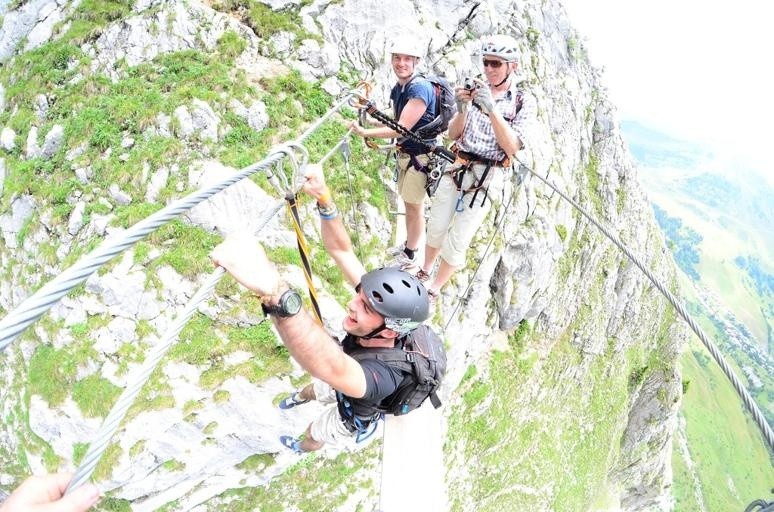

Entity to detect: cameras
[464,78,476,96]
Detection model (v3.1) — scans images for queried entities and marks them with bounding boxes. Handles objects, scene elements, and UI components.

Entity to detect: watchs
[258,281,303,320]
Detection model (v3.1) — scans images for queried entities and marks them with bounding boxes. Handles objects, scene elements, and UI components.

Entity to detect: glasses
[483,58,514,68]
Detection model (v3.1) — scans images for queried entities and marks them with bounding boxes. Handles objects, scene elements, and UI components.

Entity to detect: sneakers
[280,437,308,453]
[278,392,311,409]
[383,242,439,304]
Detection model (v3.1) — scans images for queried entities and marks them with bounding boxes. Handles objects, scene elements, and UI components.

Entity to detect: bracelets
[315,195,339,220]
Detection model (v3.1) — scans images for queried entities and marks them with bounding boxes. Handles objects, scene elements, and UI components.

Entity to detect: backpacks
[401,72,457,139]
[350,323,445,416]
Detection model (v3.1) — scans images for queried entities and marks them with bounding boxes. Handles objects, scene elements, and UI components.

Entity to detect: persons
[209,165,431,456]
[346,29,442,271]
[413,33,530,304]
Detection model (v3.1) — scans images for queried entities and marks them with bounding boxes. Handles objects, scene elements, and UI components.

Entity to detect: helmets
[356,268,430,339]
[481,35,521,63]
[389,36,424,58]
